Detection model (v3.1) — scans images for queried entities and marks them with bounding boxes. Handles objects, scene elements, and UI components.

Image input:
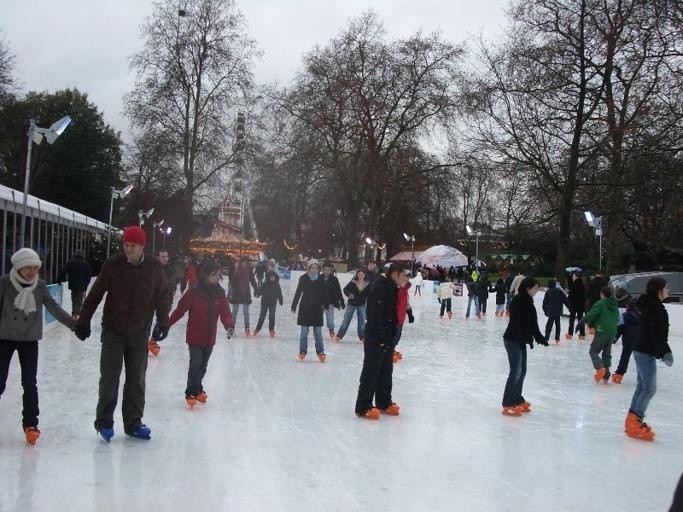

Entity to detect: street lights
[458,221,484,286]
[577,209,608,282]
[401,230,418,280]
[363,234,380,265]
[18,109,79,285]
[103,179,177,269]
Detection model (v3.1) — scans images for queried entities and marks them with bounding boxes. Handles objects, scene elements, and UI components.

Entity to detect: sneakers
[502,406,523,416]
[438,311,509,319]
[380,401,399,415]
[363,407,380,419]
[392,351,403,363]
[594,366,606,382]
[299,350,306,359]
[328,330,364,343]
[519,401,532,411]
[244,327,276,338]
[23,424,40,442]
[185,394,196,407]
[124,422,152,438]
[197,390,208,404]
[317,351,327,362]
[604,377,611,385]
[612,373,624,383]
[544,328,595,345]
[98,424,114,440]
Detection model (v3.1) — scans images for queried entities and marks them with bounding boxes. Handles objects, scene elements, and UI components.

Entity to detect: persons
[619,277,674,437]
[0,246,90,440]
[58,248,90,322]
[78,225,173,441]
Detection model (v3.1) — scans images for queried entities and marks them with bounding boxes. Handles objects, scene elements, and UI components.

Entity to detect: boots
[624,411,654,441]
[148,337,161,353]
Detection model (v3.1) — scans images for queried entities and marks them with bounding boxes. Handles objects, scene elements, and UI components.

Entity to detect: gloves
[72,316,92,341]
[226,326,235,339]
[407,310,416,324]
[662,350,674,366]
[151,324,169,339]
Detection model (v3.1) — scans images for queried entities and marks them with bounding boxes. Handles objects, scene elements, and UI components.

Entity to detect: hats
[203,259,220,274]
[122,225,146,247]
[11,247,43,270]
[306,258,321,271]
